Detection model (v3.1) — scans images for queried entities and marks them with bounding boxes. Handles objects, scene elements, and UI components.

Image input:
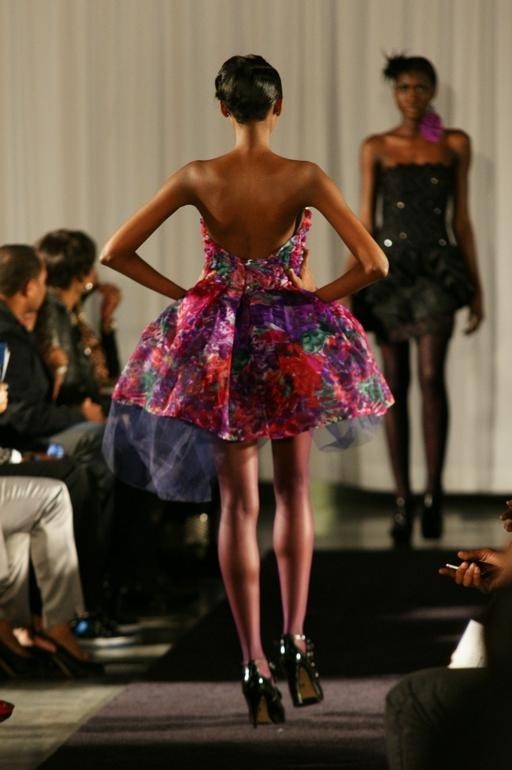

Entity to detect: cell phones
[445,559,497,576]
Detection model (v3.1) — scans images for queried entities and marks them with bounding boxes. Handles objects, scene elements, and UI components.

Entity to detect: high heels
[388,492,444,545]
[28,630,102,680]
[241,659,286,727]
[279,632,323,709]
[2,638,37,676]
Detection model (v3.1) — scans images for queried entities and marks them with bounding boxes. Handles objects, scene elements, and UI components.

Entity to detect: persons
[98,50,393,724]
[0,227,205,686]
[353,54,483,547]
[381,496,511,770]
